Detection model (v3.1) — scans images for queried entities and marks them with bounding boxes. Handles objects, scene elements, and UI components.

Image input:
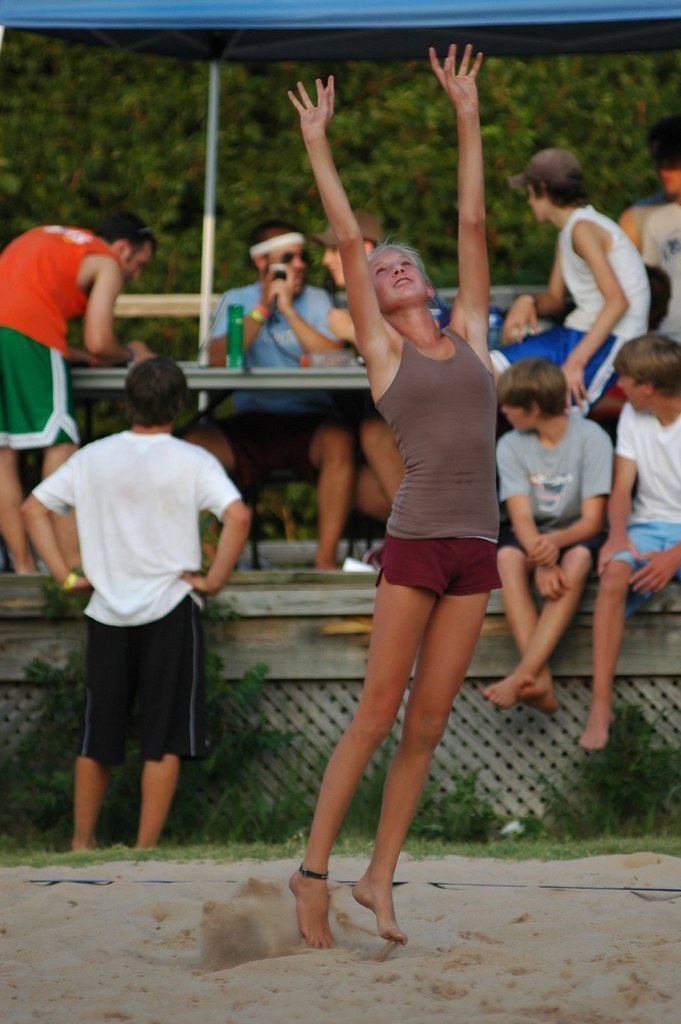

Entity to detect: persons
[190,212,449,576]
[22,355,252,851]
[288,45,504,948]
[0,213,155,577]
[491,147,651,423]
[484,356,611,712]
[577,335,681,749]
[618,116,681,346]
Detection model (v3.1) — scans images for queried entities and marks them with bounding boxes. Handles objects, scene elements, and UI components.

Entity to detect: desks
[69,363,370,409]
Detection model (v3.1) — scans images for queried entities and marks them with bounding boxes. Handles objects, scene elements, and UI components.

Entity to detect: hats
[509,149,584,189]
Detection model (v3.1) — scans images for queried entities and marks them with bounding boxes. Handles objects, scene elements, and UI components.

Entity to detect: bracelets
[60,572,77,592]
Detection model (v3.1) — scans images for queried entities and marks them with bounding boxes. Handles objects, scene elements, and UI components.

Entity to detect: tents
[0,0,681,417]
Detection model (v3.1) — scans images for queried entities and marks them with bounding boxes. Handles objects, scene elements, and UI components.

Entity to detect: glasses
[281,251,310,263]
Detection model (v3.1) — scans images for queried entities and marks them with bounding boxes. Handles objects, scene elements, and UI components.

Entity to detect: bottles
[299,351,354,368]
[487,305,503,351]
[427,296,442,330]
[226,305,245,369]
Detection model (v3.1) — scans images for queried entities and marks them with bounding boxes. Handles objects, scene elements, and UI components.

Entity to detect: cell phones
[269,264,287,281]
